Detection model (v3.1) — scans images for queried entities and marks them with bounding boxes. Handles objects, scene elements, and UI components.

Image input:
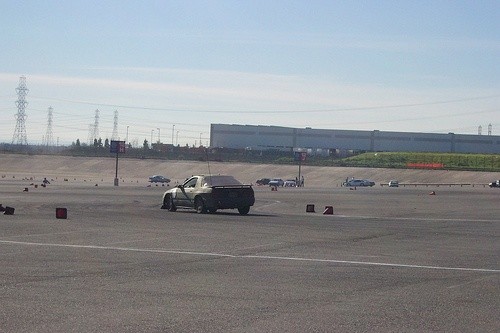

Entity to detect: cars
[269,178,284,186]
[149,175,170,184]
[162,174,255,214]
[344,178,376,186]
[489,180,500,188]
[389,180,399,187]
[256,178,270,186]
[284,179,297,187]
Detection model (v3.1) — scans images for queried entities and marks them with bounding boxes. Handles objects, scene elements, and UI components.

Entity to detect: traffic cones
[322,205,333,215]
